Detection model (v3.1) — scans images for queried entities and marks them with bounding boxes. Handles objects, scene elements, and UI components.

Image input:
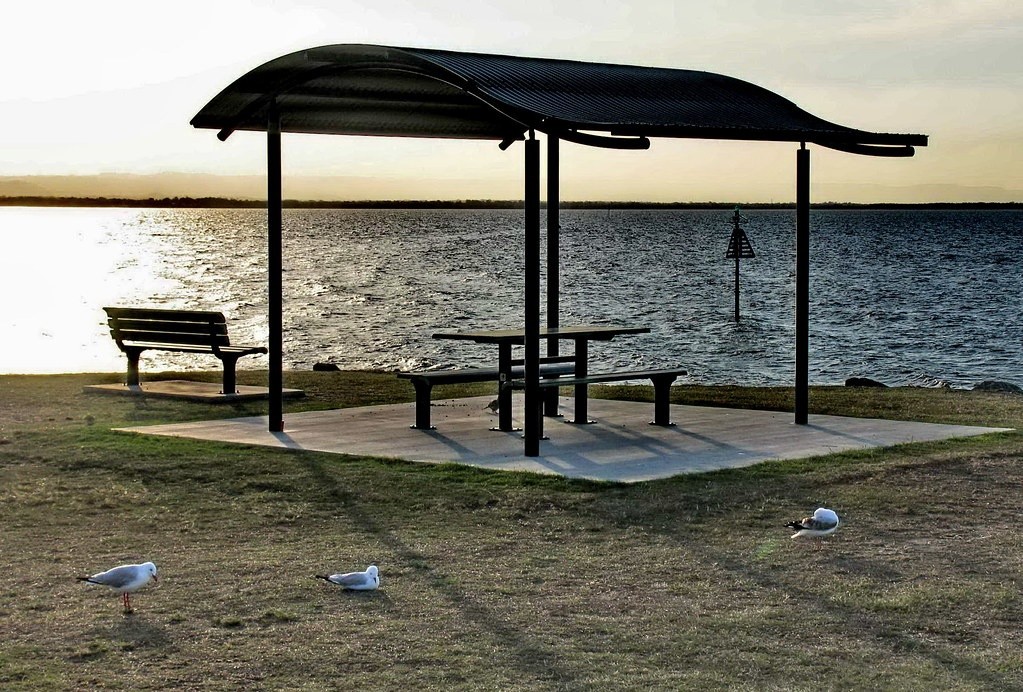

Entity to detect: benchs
[396,362,575,430]
[102,306,268,393]
[502,368,687,440]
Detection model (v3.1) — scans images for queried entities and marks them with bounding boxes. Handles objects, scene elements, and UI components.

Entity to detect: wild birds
[314,563,382,592]
[75,561,158,616]
[782,506,838,539]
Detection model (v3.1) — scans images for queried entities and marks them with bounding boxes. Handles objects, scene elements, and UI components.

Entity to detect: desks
[432,326,651,431]
